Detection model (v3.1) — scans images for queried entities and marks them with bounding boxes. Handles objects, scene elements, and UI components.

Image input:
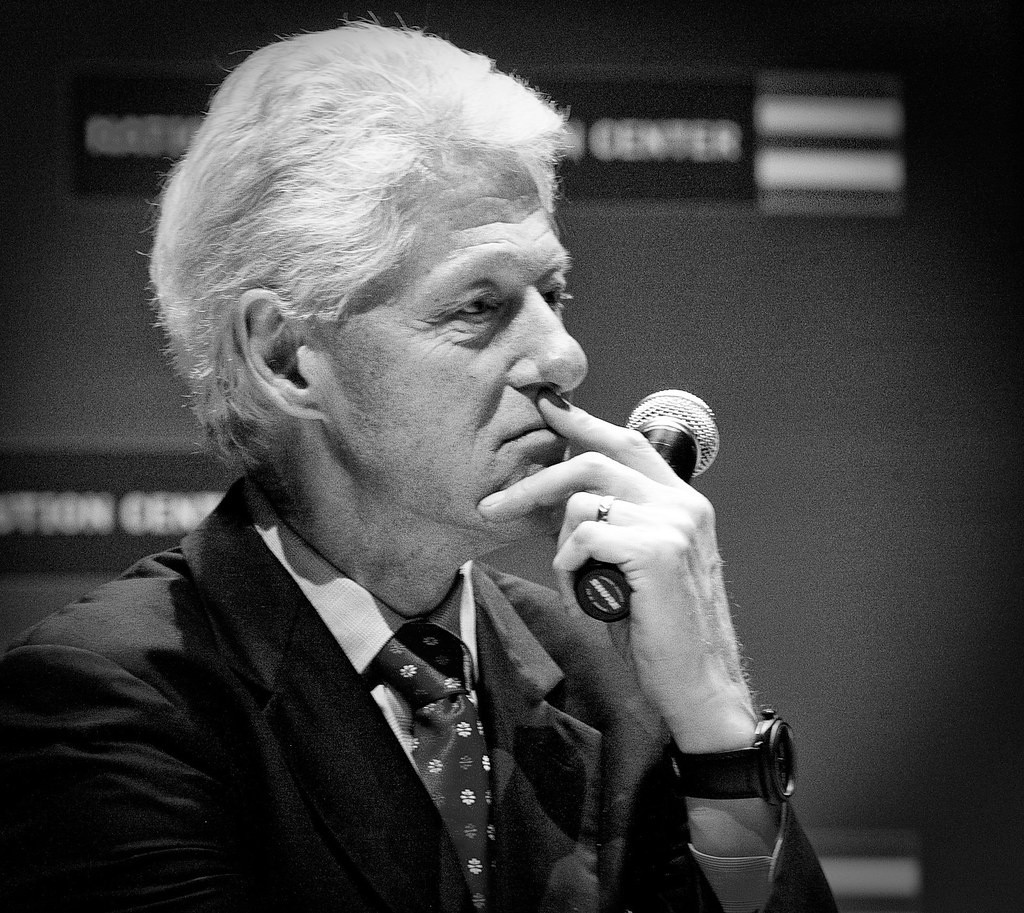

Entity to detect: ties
[365,621,496,913]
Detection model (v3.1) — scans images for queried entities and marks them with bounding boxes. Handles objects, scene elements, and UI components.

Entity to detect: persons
[0,20,837,913]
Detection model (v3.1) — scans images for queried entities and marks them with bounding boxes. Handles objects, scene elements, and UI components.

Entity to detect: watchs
[670,708,797,806]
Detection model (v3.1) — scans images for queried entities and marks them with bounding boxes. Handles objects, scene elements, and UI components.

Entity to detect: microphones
[572,389,722,621]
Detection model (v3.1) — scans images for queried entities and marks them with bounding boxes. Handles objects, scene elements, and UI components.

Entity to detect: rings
[596,493,617,522]
